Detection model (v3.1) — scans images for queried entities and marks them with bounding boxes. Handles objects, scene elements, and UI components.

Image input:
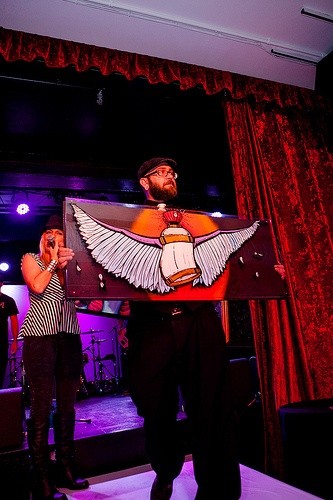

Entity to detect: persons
[21,216,89,500]
[58,158,286,500]
[0,273,19,390]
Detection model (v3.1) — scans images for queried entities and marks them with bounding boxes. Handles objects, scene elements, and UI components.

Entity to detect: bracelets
[47,259,58,273]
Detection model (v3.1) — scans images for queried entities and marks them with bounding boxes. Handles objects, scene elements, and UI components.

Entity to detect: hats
[42,215,63,229]
[138,158,176,179]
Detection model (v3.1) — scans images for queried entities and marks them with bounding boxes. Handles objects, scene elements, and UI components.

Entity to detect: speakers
[0,385,23,451]
[230,354,260,410]
[277,398,333,500]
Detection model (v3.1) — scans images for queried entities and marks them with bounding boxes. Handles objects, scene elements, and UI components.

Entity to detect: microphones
[46,233,59,262]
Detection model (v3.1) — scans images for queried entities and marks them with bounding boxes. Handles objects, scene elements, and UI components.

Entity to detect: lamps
[15,197,29,215]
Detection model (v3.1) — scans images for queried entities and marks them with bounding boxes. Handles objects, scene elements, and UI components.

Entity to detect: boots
[26,419,67,500]
[51,412,89,489]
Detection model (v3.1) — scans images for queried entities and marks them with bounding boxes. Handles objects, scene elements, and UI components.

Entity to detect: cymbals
[82,330,104,334]
[94,339,108,342]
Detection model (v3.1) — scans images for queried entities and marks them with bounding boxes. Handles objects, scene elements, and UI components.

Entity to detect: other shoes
[151,469,181,500]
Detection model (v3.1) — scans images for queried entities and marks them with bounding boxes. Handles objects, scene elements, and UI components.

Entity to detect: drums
[83,352,88,363]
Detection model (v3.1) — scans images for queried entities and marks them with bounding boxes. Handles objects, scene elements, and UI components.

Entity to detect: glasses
[144,170,178,179]
